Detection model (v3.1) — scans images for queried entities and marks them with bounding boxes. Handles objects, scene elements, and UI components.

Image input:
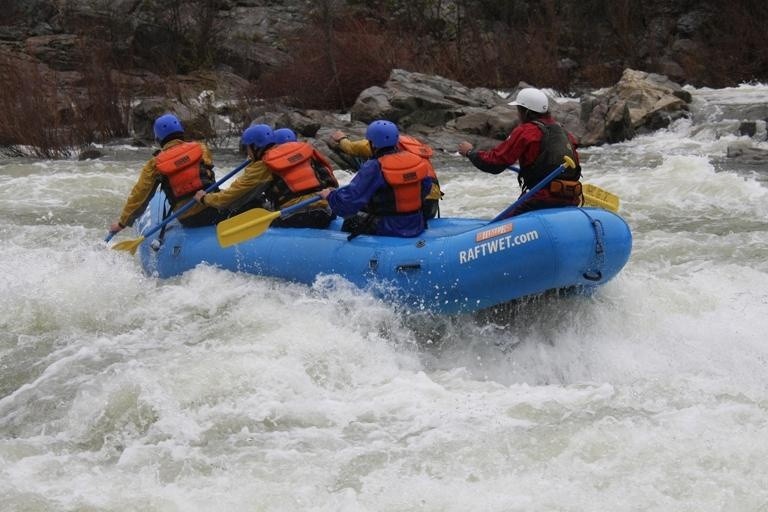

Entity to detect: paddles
[507,166,619,213]
[108,157,253,255]
[216,195,324,249]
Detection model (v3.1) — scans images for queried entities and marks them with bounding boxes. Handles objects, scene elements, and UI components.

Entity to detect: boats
[128,180,632,312]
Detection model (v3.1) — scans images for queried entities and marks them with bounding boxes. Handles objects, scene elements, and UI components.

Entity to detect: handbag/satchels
[550,179,582,197]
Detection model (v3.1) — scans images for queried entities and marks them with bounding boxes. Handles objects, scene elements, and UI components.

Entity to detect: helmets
[365,120,398,148]
[508,88,549,113]
[239,124,296,150]
[153,115,183,142]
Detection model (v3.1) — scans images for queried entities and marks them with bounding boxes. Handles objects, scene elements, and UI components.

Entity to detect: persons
[273,128,339,221]
[193,123,331,228]
[458,87,581,219]
[110,114,220,234]
[331,131,441,220]
[318,120,432,238]
